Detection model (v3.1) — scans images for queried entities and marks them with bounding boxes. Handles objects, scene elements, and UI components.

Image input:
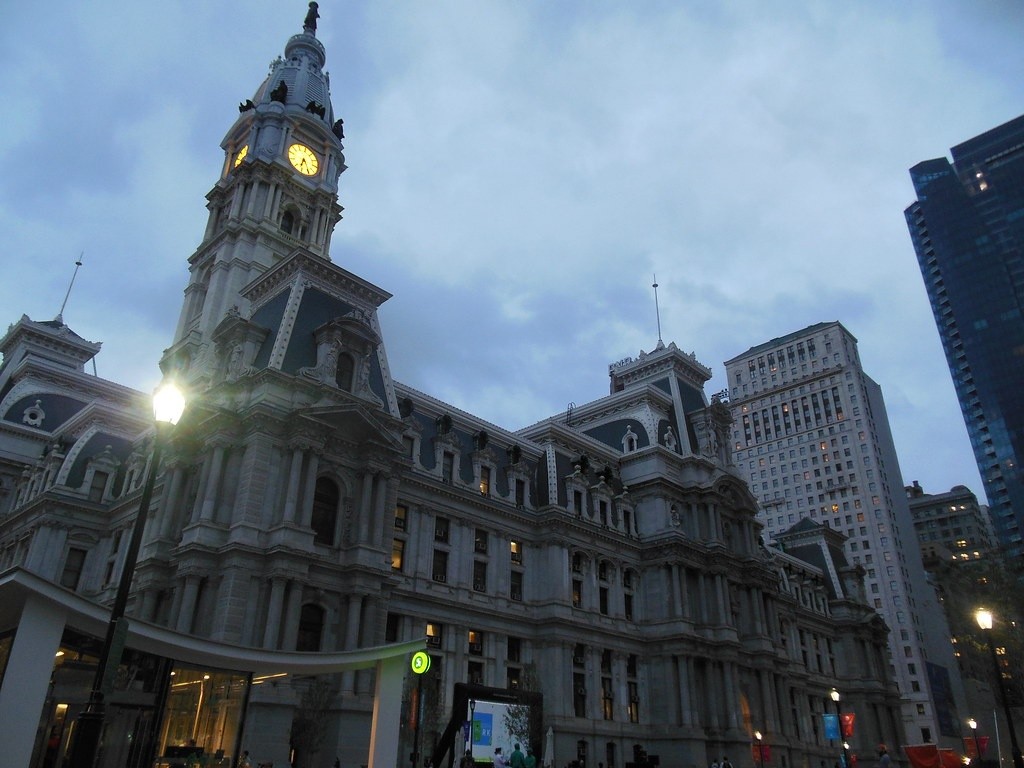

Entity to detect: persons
[709,756,734,768]
[494,743,536,768]
[874,744,891,768]
[459,749,475,768]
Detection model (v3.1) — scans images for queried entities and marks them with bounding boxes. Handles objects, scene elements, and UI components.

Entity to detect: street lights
[968,720,984,768]
[974,608,1024,768]
[755,732,768,768]
[65,381,186,768]
[829,690,853,768]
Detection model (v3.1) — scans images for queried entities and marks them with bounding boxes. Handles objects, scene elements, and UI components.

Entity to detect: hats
[879,745,886,751]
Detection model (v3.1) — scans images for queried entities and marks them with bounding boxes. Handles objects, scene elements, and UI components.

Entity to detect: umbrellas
[544,725,554,768]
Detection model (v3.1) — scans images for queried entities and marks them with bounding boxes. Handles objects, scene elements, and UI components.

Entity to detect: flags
[904,745,961,768]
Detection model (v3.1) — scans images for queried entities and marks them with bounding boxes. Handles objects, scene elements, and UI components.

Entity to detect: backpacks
[722,761,731,768]
